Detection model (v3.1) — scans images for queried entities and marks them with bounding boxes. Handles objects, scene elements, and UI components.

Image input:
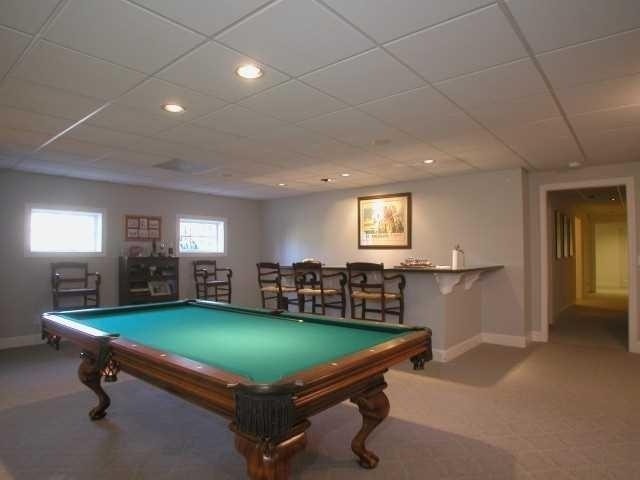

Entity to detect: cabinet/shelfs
[117,255,180,305]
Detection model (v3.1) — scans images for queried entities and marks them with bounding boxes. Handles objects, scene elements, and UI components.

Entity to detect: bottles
[151,237,175,257]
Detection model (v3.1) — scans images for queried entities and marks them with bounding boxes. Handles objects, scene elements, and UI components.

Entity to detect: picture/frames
[570,223,574,258]
[123,214,164,242]
[555,209,563,260]
[356,191,413,251]
[563,217,570,258]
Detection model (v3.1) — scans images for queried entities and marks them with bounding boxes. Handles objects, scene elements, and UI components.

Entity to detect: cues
[187,300,303,324]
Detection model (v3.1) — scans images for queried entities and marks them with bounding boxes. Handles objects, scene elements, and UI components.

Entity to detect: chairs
[347,260,408,325]
[255,260,299,311]
[193,258,233,305]
[293,262,347,319]
[50,261,102,351]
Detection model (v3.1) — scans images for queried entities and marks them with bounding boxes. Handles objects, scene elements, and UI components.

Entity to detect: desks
[276,259,506,364]
[41,299,434,479]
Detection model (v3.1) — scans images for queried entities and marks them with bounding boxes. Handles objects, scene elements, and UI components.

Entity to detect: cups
[404,255,431,266]
